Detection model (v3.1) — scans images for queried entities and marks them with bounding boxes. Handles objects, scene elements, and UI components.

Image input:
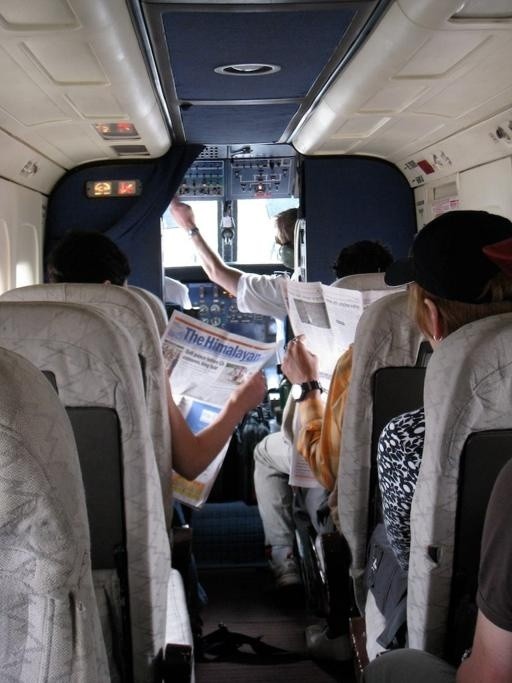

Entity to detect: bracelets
[189,228,198,236]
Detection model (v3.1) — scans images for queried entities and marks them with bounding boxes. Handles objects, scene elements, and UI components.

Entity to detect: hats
[384,210,511,305]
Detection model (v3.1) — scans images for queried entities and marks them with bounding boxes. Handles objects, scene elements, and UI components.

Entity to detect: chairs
[1,279,195,683]
[284,277,512,683]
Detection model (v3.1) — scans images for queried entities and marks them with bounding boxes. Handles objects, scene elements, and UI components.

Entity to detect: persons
[279,334,352,491]
[377,210,511,572]
[361,462,512,683]
[170,195,298,322]
[251,240,393,588]
[46,230,268,661]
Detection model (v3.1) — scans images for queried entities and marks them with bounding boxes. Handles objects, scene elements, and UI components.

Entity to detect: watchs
[291,380,323,400]
